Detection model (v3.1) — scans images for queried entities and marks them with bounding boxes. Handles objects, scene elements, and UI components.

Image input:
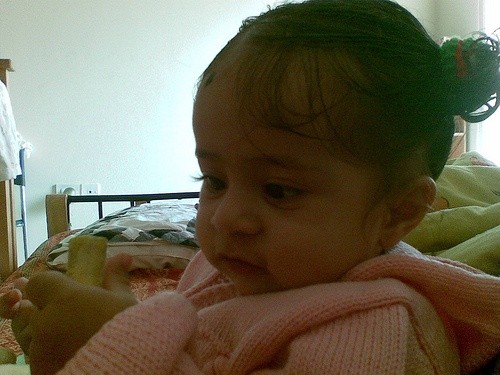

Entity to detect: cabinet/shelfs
[445,115,466,160]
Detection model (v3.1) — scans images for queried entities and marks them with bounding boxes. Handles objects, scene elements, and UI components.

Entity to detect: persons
[0,0,500,375]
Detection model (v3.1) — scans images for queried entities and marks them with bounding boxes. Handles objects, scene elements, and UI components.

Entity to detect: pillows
[45,204,200,276]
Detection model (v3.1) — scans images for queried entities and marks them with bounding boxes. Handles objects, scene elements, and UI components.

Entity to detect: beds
[0,185,201,375]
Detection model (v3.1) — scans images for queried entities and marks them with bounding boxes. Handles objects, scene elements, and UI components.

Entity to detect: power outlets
[56,185,70,195]
